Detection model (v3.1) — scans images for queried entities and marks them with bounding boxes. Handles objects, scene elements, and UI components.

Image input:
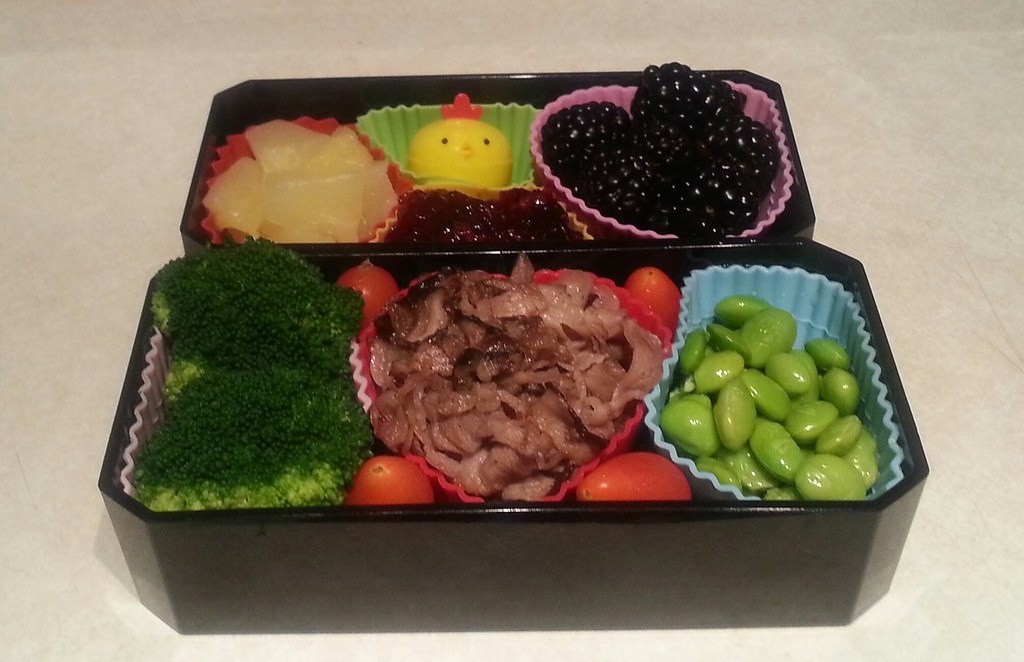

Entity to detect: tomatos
[626,267,680,342]
[577,452,691,501]
[346,456,434,504]
[337,259,398,330]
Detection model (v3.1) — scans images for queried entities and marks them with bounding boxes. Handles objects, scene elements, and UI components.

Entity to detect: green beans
[660,290,876,501]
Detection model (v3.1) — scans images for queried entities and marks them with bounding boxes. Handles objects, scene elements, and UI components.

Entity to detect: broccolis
[133,230,373,509]
